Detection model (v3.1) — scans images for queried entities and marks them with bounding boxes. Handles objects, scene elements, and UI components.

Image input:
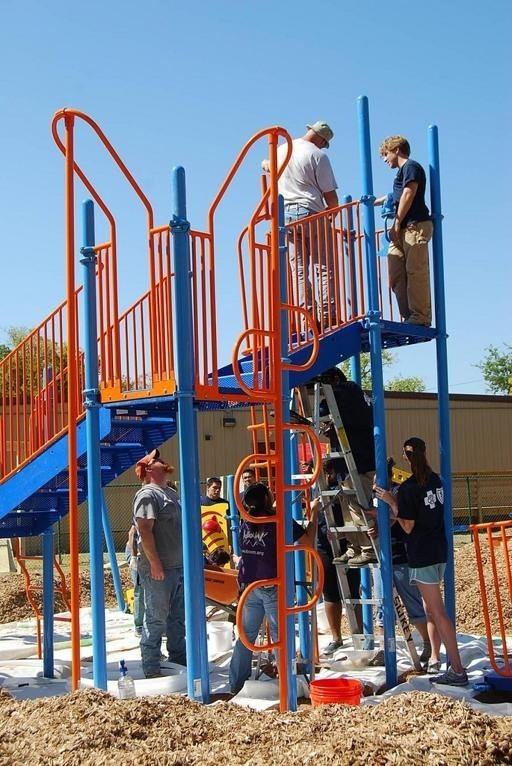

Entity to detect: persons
[262,120,339,328]
[374,136,433,326]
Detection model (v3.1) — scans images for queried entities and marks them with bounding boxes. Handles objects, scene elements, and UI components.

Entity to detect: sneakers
[370,649,388,666]
[420,639,432,662]
[427,658,441,674]
[429,666,469,685]
[323,640,343,655]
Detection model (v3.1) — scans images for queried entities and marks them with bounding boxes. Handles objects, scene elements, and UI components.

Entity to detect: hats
[306,121,334,149]
[135,448,160,481]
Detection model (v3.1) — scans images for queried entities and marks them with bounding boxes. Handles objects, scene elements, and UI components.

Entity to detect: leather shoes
[332,553,379,567]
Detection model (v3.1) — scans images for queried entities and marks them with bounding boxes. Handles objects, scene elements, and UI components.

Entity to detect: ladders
[298,378,423,672]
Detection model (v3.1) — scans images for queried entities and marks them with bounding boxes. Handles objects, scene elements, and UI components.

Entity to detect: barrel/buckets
[308,677,363,708]
